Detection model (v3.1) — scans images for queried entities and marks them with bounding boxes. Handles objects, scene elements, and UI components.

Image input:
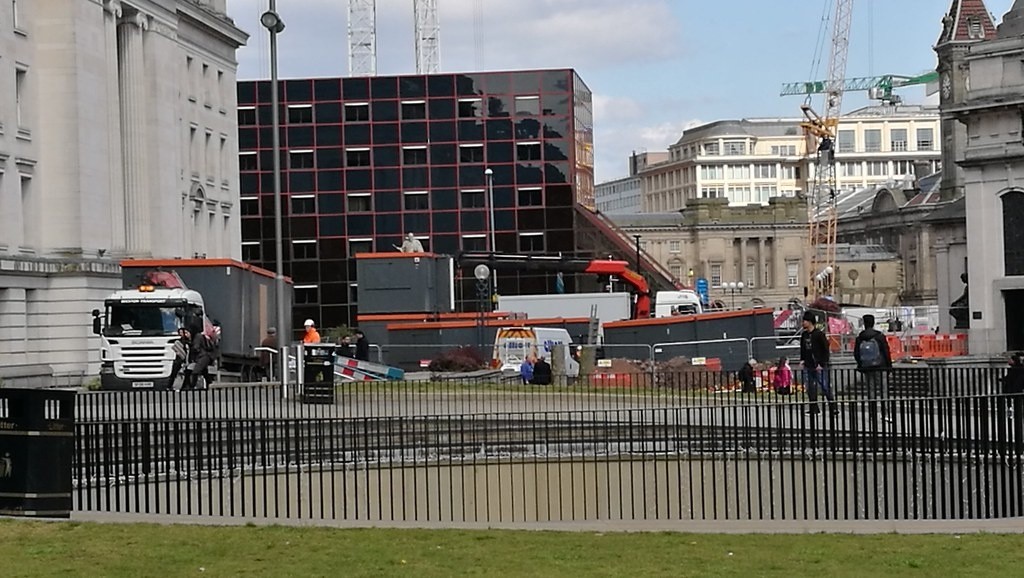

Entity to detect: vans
[493,327,580,385]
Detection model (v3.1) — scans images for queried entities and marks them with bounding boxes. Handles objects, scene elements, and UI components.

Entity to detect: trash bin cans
[0,387,78,518]
[304,346,335,403]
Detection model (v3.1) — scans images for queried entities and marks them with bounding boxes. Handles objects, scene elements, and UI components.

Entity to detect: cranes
[778,1,917,352]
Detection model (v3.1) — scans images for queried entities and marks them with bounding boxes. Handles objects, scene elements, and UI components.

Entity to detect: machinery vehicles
[458,251,650,319]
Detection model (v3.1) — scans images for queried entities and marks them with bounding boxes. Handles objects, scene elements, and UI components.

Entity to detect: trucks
[91,259,294,392]
[497,290,702,328]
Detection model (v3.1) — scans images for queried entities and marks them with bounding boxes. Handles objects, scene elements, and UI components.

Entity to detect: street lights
[721,281,745,314]
[484,169,499,304]
[631,233,641,272]
[474,264,491,368]
[259,0,288,385]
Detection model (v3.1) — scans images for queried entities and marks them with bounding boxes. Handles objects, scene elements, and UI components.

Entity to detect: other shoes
[834,410,840,415]
[881,417,893,422]
[805,409,821,416]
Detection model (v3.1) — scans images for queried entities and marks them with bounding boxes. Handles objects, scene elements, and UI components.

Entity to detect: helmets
[303,319,314,326]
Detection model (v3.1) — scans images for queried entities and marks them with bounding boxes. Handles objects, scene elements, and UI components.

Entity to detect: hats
[803,311,817,325]
[267,327,276,334]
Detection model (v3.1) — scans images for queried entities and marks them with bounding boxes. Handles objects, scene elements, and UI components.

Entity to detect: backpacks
[859,339,882,367]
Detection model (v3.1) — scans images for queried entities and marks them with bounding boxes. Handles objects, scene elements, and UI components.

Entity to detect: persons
[773,356,794,395]
[164,327,219,393]
[300,319,324,382]
[521,355,551,385]
[799,311,840,417]
[818,134,831,150]
[261,326,280,381]
[951,273,968,307]
[396,232,424,253]
[998,355,1024,393]
[335,329,370,372]
[854,314,893,422]
[887,316,904,332]
[738,358,757,393]
